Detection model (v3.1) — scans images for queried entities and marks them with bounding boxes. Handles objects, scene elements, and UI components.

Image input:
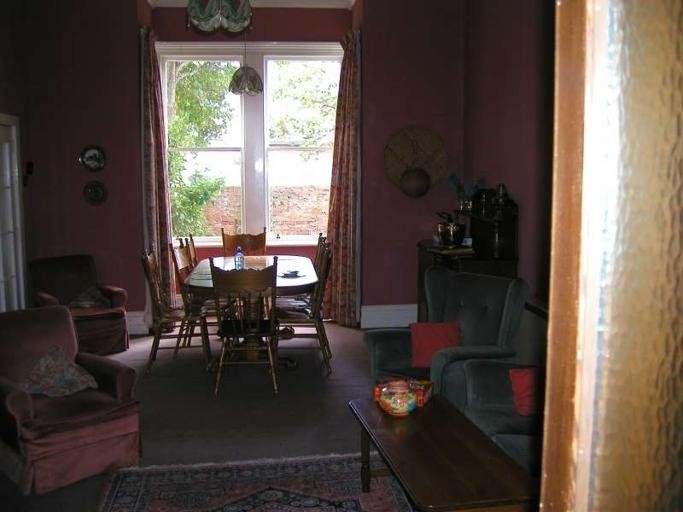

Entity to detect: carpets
[95,451,415,512]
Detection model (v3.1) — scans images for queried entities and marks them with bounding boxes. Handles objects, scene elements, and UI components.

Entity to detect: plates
[282,272,297,275]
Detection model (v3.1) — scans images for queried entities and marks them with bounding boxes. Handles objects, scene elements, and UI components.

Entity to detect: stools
[360,304,418,379]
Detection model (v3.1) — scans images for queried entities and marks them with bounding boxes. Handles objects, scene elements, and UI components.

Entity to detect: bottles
[432,183,509,248]
[234,246,244,270]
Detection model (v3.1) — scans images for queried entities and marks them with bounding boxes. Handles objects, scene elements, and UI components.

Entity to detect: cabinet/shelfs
[415,240,475,322]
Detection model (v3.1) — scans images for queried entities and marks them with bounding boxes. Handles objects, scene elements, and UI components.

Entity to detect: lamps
[185,0,252,35]
[229,27,264,97]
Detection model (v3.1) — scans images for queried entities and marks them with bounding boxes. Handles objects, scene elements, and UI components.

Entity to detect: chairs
[142,226,334,400]
[27,253,129,357]
[0,304,143,495]
[440,357,545,483]
[362,262,531,396]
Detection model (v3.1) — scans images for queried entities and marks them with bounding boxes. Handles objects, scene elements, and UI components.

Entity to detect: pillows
[409,322,461,369]
[508,367,537,418]
[22,343,99,397]
[68,286,112,309]
